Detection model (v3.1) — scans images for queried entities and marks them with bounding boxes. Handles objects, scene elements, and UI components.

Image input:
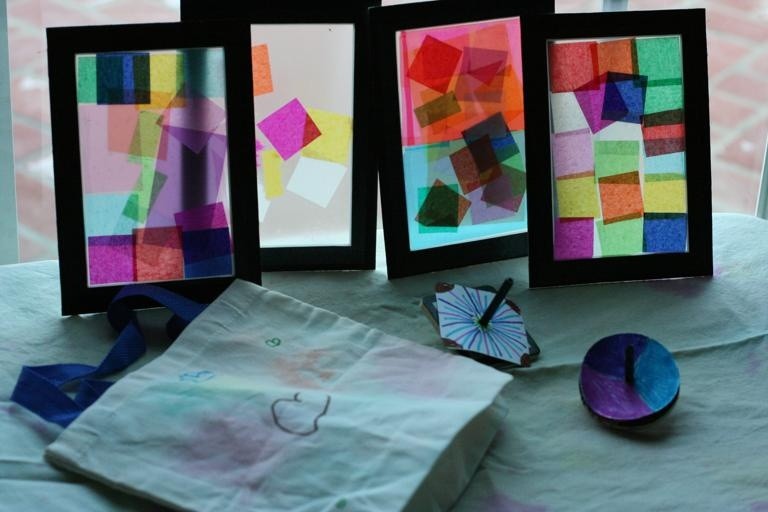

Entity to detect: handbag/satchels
[10,278,513,512]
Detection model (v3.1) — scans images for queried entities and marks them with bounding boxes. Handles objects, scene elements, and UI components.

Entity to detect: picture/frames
[524,6,715,290]
[47,20,269,320]
[360,3,556,284]
[178,1,383,274]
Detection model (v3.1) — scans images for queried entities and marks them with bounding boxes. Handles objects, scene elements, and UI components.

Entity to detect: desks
[0,208,767,512]
